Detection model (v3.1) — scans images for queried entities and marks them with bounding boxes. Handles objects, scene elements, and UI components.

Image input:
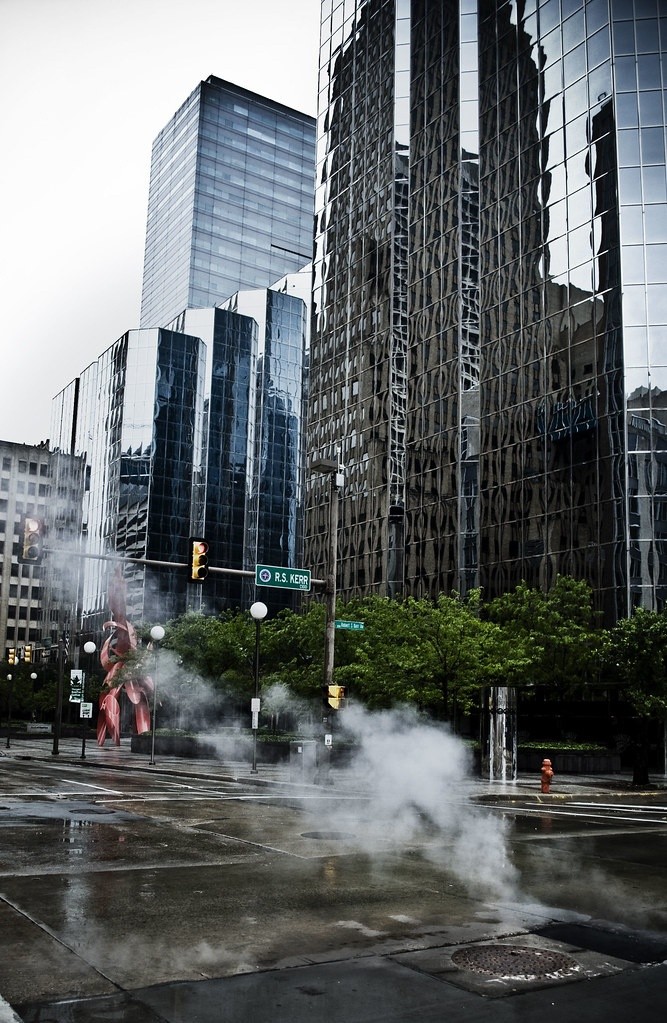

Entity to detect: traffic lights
[23,518,40,560]
[322,682,345,712]
[23,644,32,663]
[192,541,209,581]
[8,648,15,665]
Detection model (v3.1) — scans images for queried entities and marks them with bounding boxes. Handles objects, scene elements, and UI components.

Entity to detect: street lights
[81,642,97,758]
[308,457,338,789]
[30,672,37,722]
[148,625,165,766]
[4,673,15,748]
[251,602,267,772]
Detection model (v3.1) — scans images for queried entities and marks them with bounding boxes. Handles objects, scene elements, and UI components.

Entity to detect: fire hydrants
[540,758,554,794]
